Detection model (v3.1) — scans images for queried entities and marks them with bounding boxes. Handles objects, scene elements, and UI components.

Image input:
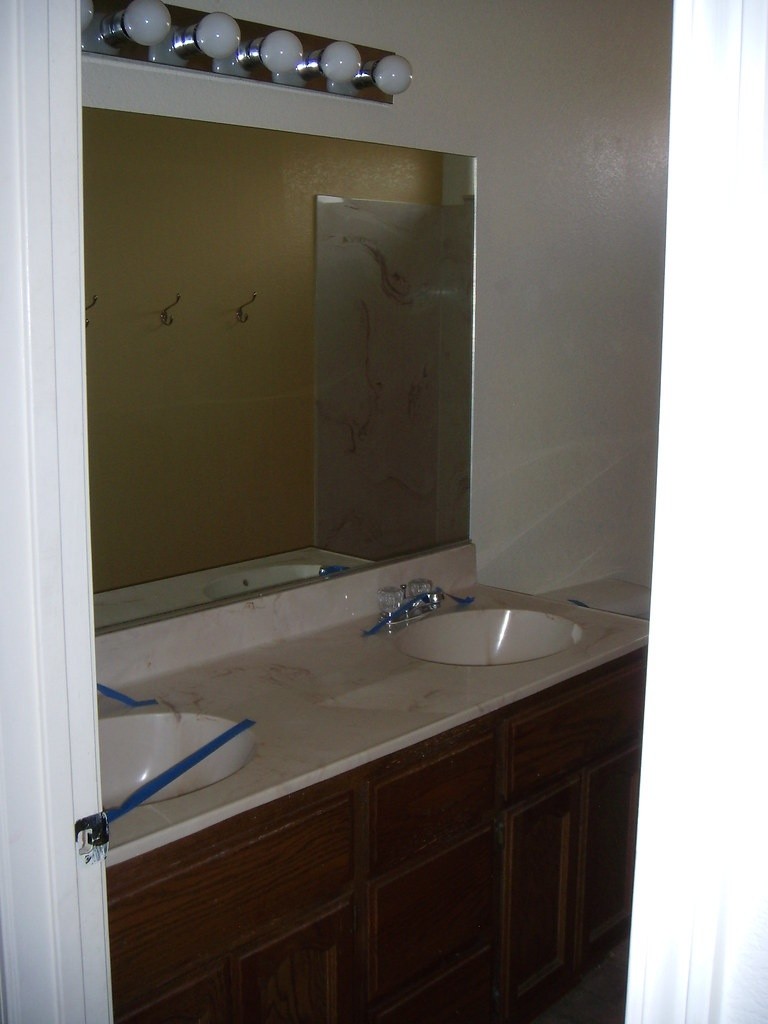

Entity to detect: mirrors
[83,105,477,639]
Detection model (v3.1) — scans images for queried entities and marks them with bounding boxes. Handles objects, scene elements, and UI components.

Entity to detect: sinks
[207,564,324,595]
[393,608,584,667]
[98,710,258,813]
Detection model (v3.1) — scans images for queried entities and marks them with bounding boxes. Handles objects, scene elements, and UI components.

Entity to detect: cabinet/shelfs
[104,646,647,1024]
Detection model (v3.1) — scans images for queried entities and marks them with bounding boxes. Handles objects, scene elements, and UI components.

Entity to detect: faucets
[399,583,445,612]
